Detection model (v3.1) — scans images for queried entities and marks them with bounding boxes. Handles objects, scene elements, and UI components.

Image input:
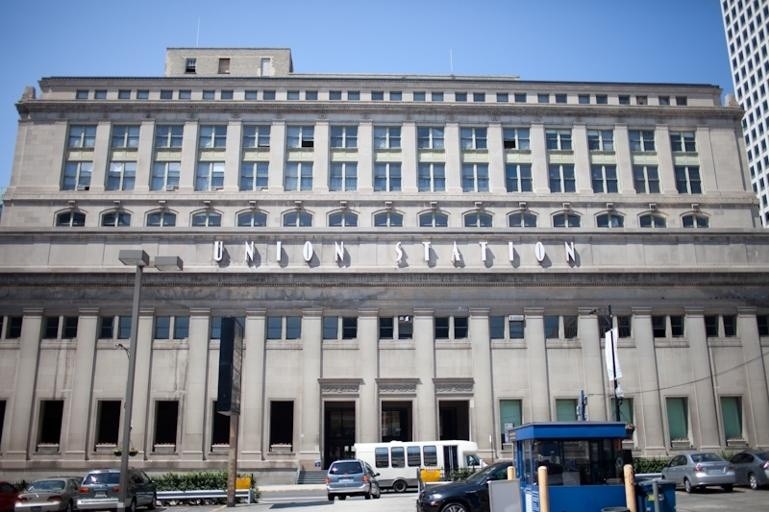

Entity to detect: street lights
[117,250,183,512]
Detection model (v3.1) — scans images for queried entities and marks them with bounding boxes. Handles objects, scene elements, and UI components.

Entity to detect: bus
[354,440,488,493]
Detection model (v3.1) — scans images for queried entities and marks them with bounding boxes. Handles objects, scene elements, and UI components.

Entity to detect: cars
[416,461,608,512]
[661,450,769,493]
[0,466,157,512]
[325,460,380,501]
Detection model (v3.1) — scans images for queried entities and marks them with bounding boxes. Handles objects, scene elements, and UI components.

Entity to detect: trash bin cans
[636,480,676,512]
